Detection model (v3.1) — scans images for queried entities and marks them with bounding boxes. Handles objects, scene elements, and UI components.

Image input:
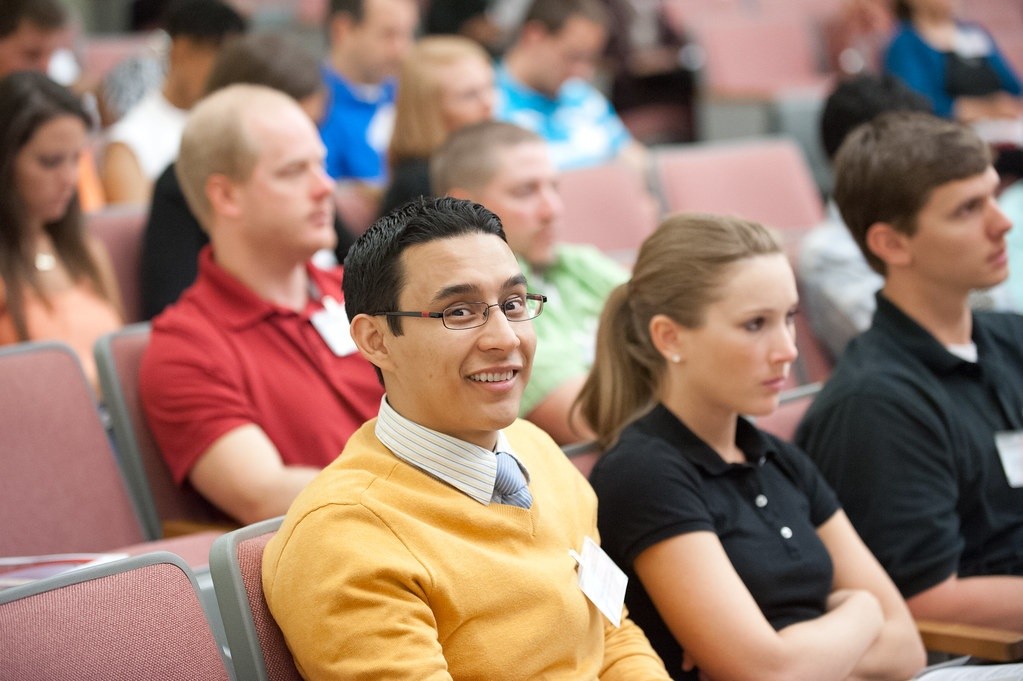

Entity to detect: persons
[568,213,927,681]
[883,1,1022,178]
[820,0,942,157]
[261,197,675,681]
[137,86,387,527]
[807,75,1023,363]
[0,68,133,510]
[428,122,633,445]
[0,0,652,320]
[792,110,1023,665]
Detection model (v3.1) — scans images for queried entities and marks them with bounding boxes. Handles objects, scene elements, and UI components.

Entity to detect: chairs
[0,0,1023,681]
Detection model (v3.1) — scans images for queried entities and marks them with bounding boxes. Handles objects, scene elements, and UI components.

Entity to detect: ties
[492,452,532,509]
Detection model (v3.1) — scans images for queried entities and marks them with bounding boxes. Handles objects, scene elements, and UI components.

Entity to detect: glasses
[370,291,547,330]
